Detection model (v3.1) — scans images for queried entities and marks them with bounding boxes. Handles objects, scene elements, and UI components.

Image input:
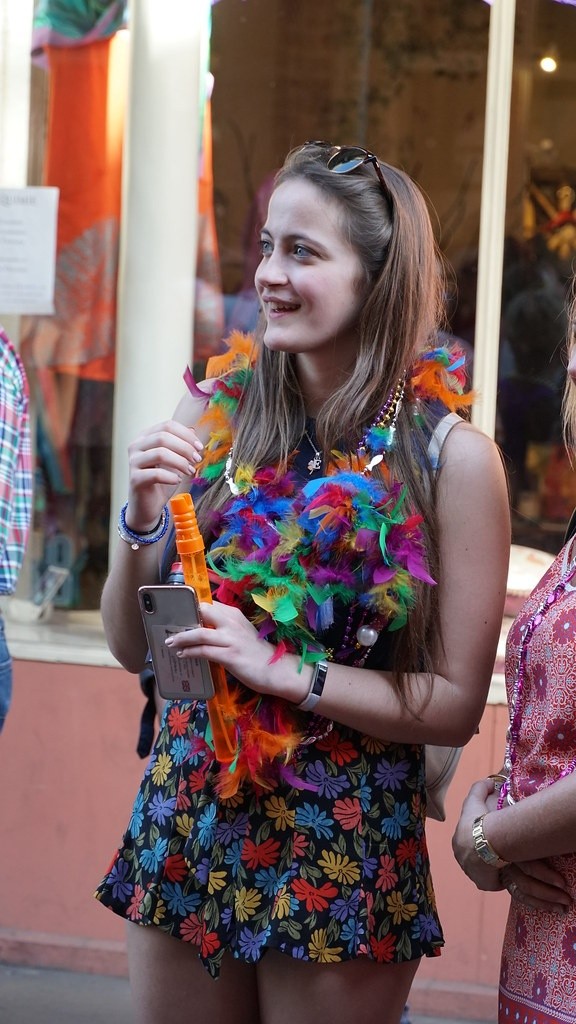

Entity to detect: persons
[446,303,576,1024]
[93,140,511,1024]
[0,323,34,733]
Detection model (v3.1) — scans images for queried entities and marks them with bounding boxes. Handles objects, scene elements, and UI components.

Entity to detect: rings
[510,883,517,893]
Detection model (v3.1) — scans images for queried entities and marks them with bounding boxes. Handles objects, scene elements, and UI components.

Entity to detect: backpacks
[420,411,466,823]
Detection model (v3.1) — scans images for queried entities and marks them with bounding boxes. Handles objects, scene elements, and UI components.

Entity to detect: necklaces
[223,378,406,759]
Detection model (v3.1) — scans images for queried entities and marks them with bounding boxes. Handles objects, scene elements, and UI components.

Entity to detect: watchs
[295,659,328,712]
[472,813,511,868]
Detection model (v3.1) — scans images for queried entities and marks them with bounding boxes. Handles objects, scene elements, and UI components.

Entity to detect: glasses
[298,139,394,224]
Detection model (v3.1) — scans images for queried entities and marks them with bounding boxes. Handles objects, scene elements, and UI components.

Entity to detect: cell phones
[137,585,214,700]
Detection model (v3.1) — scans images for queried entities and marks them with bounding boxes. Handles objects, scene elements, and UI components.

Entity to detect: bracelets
[487,774,507,782]
[117,501,170,551]
